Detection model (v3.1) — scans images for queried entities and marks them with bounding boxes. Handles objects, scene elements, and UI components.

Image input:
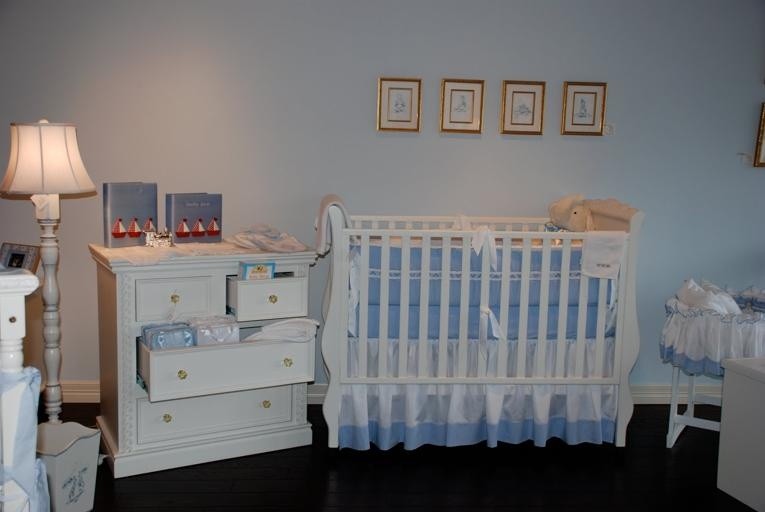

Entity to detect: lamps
[0,118,99,426]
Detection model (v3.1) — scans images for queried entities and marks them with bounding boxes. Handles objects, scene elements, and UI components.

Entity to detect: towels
[313,194,357,257]
[580,230,627,280]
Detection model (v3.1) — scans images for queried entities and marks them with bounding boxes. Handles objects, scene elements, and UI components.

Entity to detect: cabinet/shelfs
[86,240,317,478]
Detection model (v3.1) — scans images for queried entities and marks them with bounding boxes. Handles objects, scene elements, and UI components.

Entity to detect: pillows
[677,279,742,315]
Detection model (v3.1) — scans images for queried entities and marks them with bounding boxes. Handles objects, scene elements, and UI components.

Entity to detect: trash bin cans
[37,421,103,512]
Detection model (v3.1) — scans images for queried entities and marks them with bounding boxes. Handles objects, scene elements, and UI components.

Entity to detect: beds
[320,197,644,448]
[663,278,764,450]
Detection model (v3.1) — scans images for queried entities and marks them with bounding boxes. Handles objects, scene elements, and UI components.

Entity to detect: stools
[716,357,765,512]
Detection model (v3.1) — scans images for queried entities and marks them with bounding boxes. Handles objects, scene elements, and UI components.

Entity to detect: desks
[0,267,49,512]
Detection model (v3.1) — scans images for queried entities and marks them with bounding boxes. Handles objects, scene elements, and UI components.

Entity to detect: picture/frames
[0,243,41,275]
[560,81,608,136]
[376,77,422,133]
[500,79,547,135]
[440,77,485,137]
[753,102,765,167]
